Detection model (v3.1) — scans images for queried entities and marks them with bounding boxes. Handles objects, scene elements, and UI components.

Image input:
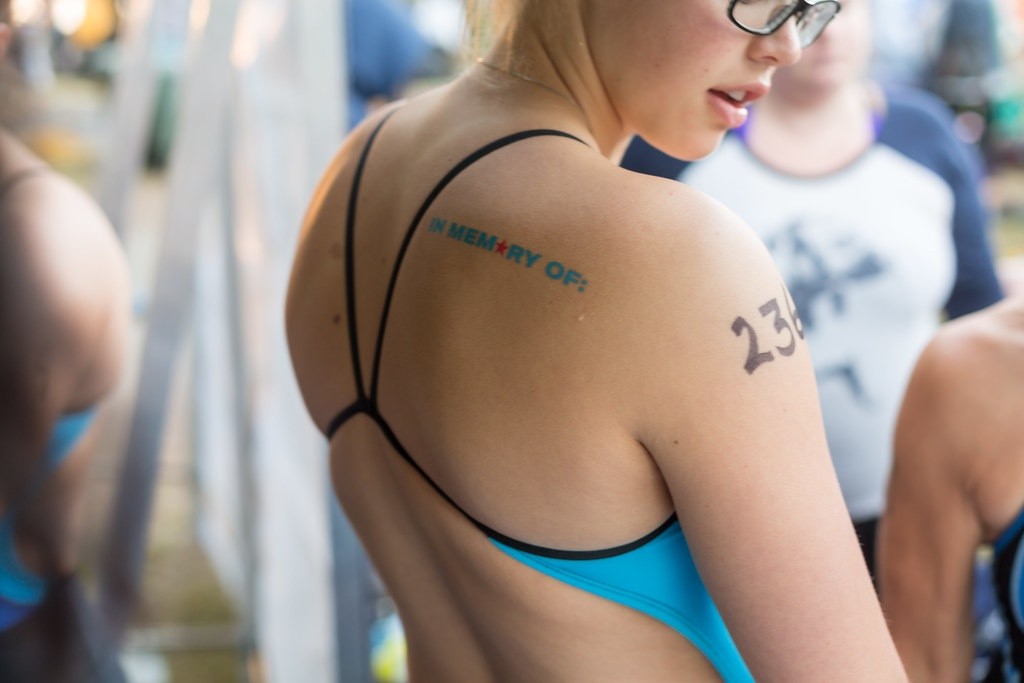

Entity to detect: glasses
[728,0,842,50]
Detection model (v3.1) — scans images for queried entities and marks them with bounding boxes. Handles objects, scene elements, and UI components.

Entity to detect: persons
[623,0,1008,564]
[863,290,1024,683]
[2,126,130,683]
[281,0,914,683]
